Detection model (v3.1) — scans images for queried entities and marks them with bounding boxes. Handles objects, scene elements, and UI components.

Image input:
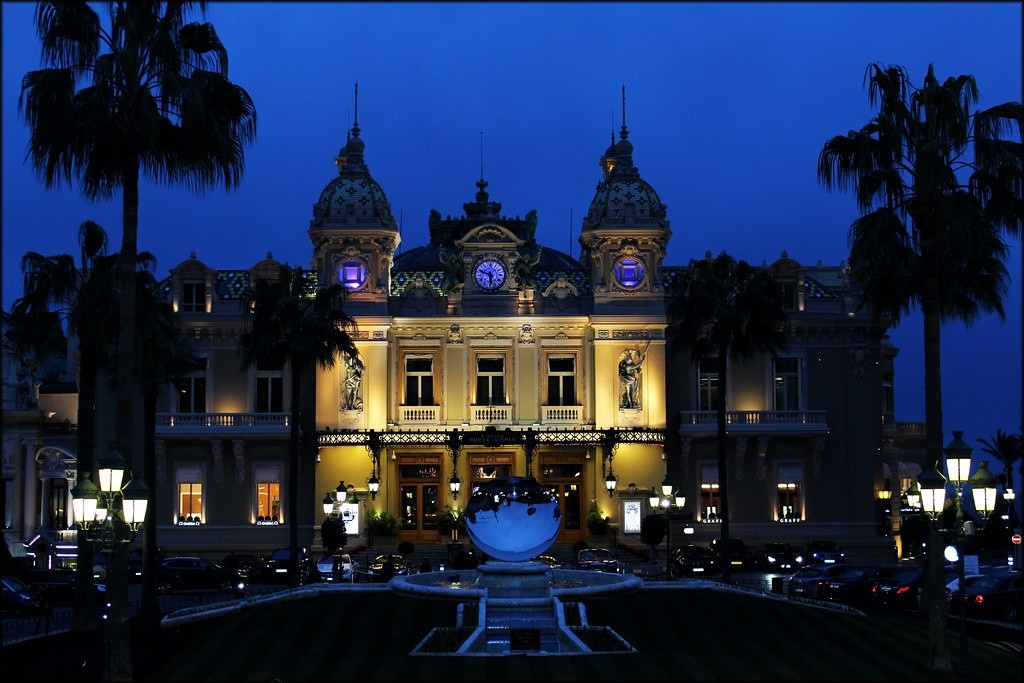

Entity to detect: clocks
[471,257,510,294]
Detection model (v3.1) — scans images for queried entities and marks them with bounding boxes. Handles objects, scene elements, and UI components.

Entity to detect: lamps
[365,439,386,500]
[317,455,321,462]
[445,440,464,500]
[391,451,397,459]
[586,451,590,459]
[601,438,619,498]
[661,454,665,460]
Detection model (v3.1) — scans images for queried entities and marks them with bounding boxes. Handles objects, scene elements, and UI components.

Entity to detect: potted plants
[584,511,612,546]
[366,510,404,546]
[435,509,467,545]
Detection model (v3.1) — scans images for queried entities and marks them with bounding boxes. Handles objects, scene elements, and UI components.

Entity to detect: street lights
[68,444,152,683]
[920,430,997,676]
[322,480,360,583]
[649,474,685,581]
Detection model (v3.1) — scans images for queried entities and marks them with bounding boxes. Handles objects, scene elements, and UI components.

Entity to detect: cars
[217,544,315,587]
[789,564,864,599]
[848,561,928,611]
[0,567,76,618]
[314,553,357,583]
[160,557,221,589]
[671,544,716,576]
[706,537,752,573]
[570,548,618,573]
[366,554,409,584]
[757,542,802,575]
[898,566,1024,646]
[805,538,846,565]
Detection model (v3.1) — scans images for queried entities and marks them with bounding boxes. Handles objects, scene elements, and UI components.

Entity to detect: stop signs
[1012,534,1022,544]
